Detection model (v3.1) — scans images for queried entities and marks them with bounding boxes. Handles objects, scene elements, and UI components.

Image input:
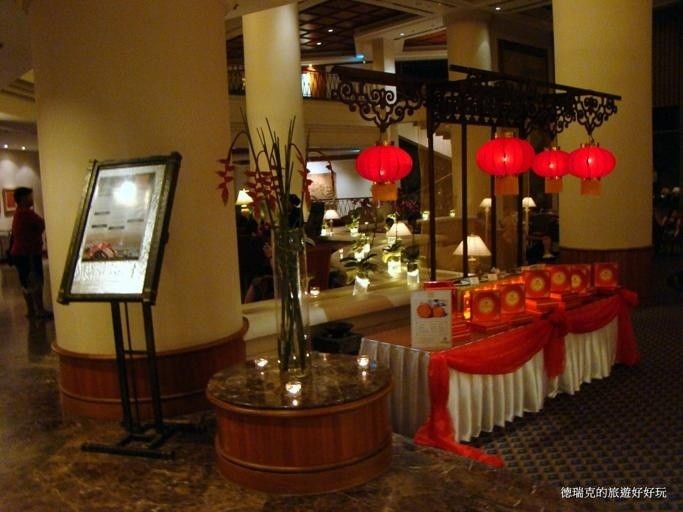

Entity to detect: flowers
[211,110,337,375]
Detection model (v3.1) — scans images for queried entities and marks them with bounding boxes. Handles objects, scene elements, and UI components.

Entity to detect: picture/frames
[1,188,17,215]
[56,150,186,305]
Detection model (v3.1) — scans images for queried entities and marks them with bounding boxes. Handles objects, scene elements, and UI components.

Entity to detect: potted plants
[337,212,423,300]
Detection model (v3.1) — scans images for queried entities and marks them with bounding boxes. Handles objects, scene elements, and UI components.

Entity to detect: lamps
[322,208,339,232]
[451,235,492,276]
[235,189,254,217]
[522,197,537,245]
[480,198,491,246]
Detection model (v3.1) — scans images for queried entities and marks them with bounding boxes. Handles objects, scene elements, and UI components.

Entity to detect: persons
[4,185,54,320]
[384,216,413,236]
[235,192,304,303]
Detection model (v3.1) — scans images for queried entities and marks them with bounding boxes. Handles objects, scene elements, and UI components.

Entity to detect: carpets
[468,303,683,511]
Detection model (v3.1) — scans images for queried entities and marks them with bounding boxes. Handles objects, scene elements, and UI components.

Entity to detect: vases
[265,218,313,375]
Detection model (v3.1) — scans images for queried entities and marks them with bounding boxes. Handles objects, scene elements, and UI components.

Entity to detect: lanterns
[473,127,537,196]
[352,138,412,205]
[525,142,569,196]
[568,138,616,196]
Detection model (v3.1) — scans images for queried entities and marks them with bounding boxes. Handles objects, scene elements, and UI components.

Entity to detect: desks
[359,284,637,443]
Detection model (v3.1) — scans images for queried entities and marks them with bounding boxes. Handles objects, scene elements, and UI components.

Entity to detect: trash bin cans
[313,322,361,355]
